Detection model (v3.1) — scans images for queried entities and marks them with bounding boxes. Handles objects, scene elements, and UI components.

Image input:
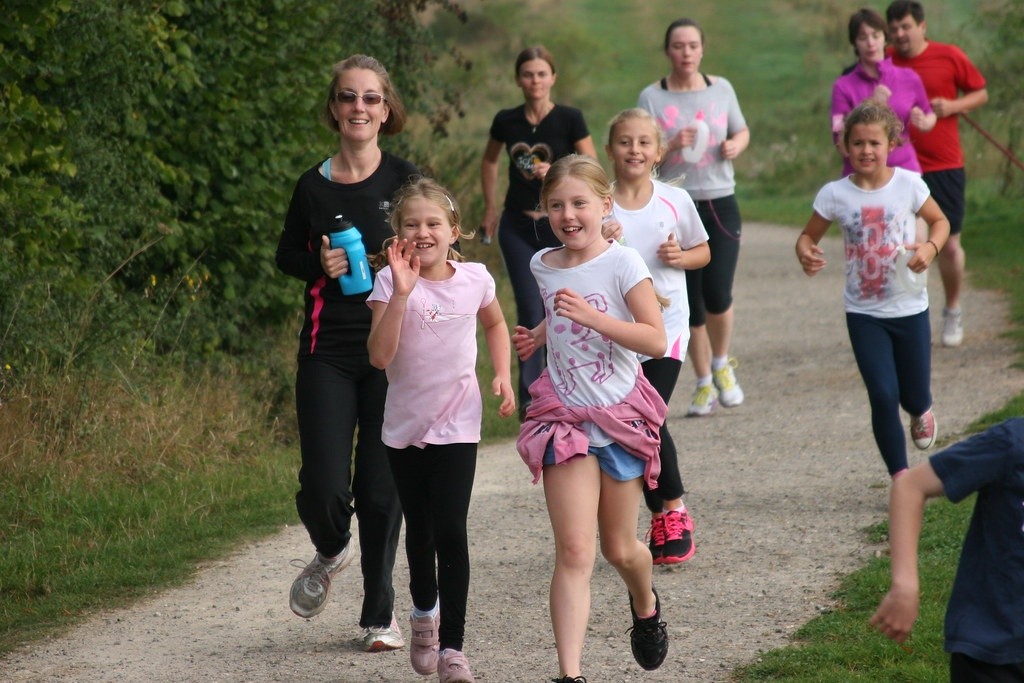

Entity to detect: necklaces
[526,102,551,134]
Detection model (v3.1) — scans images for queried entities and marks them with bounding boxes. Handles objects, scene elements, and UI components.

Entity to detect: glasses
[334,91,388,105]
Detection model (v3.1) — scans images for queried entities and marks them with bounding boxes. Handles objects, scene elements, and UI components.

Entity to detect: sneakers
[437,648,475,683]
[941,305,963,346]
[360,610,404,652]
[907,407,938,450]
[664,507,696,563]
[687,377,719,416]
[712,357,744,407]
[644,511,667,565]
[409,604,440,675]
[552,674,587,683]
[289,536,357,618]
[625,585,669,670]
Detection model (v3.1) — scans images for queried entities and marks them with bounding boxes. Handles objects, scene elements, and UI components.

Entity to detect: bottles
[601,210,628,248]
[896,245,928,298]
[680,109,710,163]
[327,214,373,295]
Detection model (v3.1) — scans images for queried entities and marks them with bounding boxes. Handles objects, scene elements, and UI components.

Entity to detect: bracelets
[927,241,938,257]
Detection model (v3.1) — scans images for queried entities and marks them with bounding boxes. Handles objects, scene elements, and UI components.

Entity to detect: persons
[868,419,1024,657]
[884,0,989,347]
[636,18,749,417]
[510,152,669,683]
[275,55,424,653]
[478,46,598,425]
[832,8,938,180]
[366,179,515,683]
[794,102,951,484]
[602,109,711,567]
